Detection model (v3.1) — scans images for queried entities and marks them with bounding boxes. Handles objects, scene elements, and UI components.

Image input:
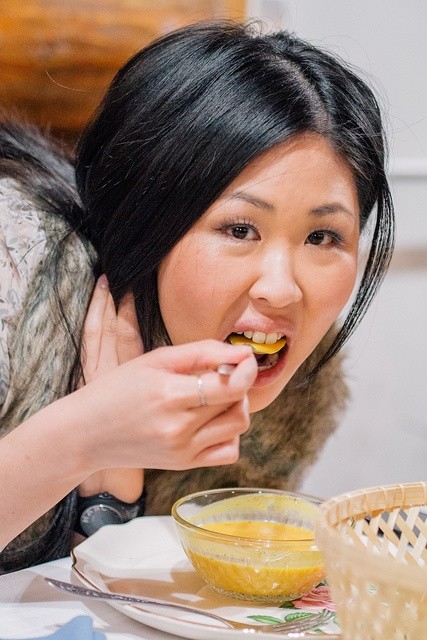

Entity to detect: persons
[0,25,399,573]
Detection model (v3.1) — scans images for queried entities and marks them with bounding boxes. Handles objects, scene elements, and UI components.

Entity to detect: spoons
[217,335,288,377]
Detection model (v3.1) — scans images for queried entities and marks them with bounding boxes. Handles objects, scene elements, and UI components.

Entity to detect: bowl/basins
[170,487,339,602]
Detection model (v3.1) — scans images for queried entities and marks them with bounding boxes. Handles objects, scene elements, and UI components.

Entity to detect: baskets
[314,480,427,640]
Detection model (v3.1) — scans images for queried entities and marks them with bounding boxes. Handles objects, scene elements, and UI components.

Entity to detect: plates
[70,515,339,640]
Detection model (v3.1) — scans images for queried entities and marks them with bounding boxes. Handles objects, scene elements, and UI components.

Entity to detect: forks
[45,578,334,636]
